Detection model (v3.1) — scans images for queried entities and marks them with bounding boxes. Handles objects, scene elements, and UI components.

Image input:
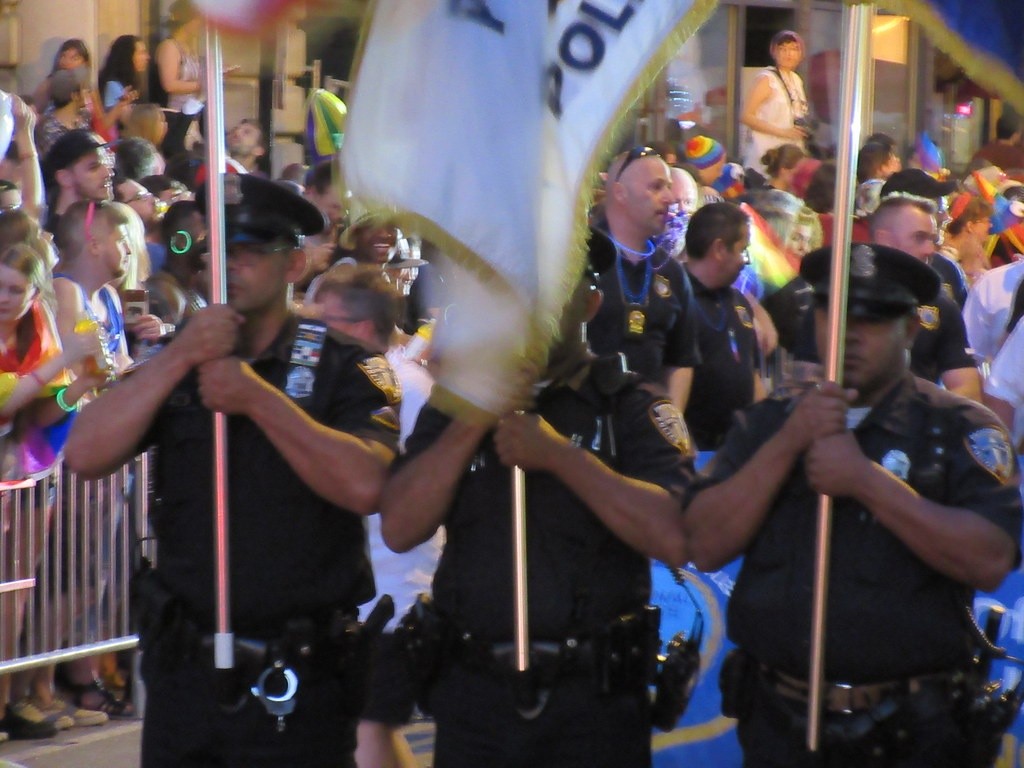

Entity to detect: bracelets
[19,151,39,161]
[55,389,76,412]
[29,371,45,387]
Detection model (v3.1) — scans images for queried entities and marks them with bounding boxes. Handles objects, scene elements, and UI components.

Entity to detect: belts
[761,666,983,712]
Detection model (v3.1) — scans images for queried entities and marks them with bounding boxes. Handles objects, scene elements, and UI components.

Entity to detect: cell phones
[124,289,148,332]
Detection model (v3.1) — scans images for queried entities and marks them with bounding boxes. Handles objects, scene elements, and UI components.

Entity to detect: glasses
[124,191,152,204]
[615,145,662,182]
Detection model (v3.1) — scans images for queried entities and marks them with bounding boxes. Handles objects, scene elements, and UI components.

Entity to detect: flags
[333,0,722,429]
[854,0,1024,120]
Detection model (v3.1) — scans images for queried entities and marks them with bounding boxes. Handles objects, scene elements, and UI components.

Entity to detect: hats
[964,165,1023,195]
[195,172,324,255]
[800,241,941,320]
[586,226,618,282]
[683,135,724,169]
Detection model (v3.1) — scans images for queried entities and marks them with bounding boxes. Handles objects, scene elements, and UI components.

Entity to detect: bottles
[74,309,117,397]
[405,319,437,360]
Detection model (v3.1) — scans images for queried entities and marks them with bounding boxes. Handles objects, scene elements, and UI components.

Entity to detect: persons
[376,255,694,767]
[193,116,267,190]
[580,146,701,412]
[61,176,402,767]
[795,189,984,404]
[97,33,151,127]
[684,243,1020,768]
[1,37,1024,748]
[739,29,811,175]
[148,0,207,159]
[678,202,767,451]
